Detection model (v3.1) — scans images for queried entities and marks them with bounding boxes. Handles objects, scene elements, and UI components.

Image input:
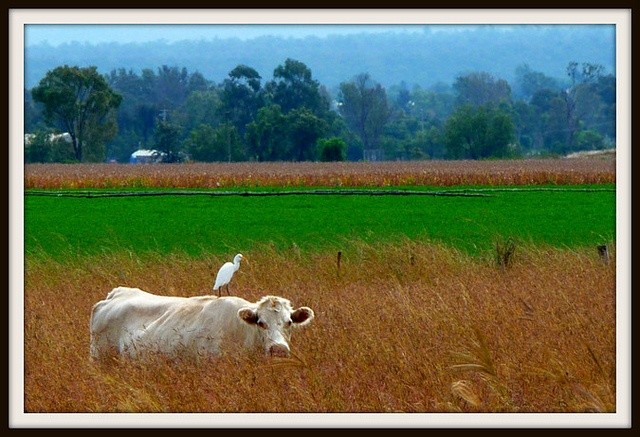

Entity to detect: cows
[89,285,315,370]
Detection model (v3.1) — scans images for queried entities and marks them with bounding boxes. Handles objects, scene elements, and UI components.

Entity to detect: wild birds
[214,253,250,296]
